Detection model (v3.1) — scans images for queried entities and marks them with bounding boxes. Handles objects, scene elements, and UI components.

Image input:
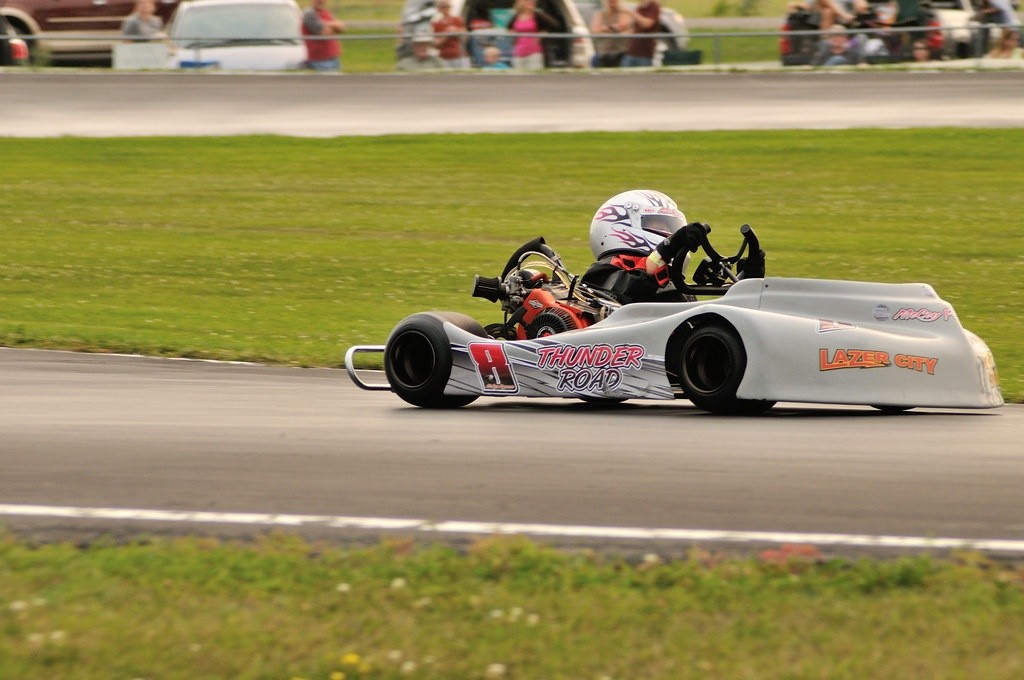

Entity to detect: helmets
[588,190,687,261]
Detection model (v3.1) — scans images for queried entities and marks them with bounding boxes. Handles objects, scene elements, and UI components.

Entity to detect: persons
[576,187,709,330]
[480,47,511,70]
[622,1,661,68]
[117,0,166,44]
[431,0,472,70]
[504,0,559,72]
[786,0,1022,67]
[590,0,636,69]
[299,0,349,74]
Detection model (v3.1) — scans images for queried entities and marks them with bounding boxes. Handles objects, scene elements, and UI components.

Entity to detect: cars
[164,2,308,74]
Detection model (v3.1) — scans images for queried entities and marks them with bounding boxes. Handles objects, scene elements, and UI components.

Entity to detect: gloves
[656,222,707,263]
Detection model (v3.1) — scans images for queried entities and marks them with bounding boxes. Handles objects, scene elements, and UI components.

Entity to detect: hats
[411,22,434,43]
